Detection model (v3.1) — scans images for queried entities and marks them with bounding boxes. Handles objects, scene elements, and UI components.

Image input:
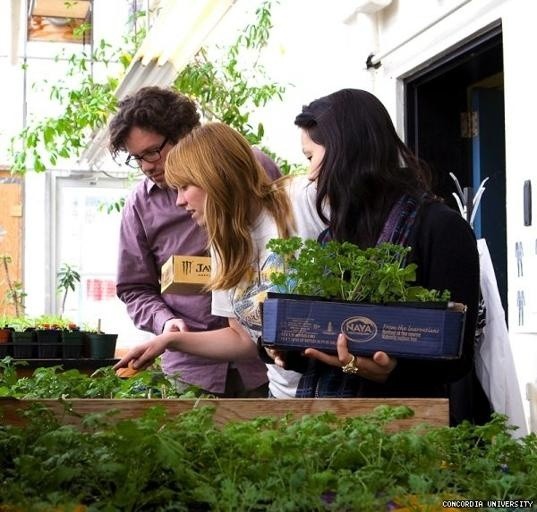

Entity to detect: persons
[105,85,281,398]
[107,120,333,398]
[254,88,497,428]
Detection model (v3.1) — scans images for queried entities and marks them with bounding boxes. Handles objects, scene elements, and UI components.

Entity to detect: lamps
[78,0,235,166]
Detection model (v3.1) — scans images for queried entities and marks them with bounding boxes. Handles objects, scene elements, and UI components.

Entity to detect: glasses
[122,137,171,169]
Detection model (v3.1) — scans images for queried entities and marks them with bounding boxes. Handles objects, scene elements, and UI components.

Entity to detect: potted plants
[0,321,12,358]
[12,324,36,359]
[88,333,117,358]
[264,236,451,309]
[63,323,85,357]
[36,323,64,358]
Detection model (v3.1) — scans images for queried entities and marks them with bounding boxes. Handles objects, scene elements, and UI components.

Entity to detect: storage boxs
[162,255,210,296]
[260,298,467,361]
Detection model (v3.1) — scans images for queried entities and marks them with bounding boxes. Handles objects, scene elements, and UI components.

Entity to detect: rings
[341,353,359,375]
